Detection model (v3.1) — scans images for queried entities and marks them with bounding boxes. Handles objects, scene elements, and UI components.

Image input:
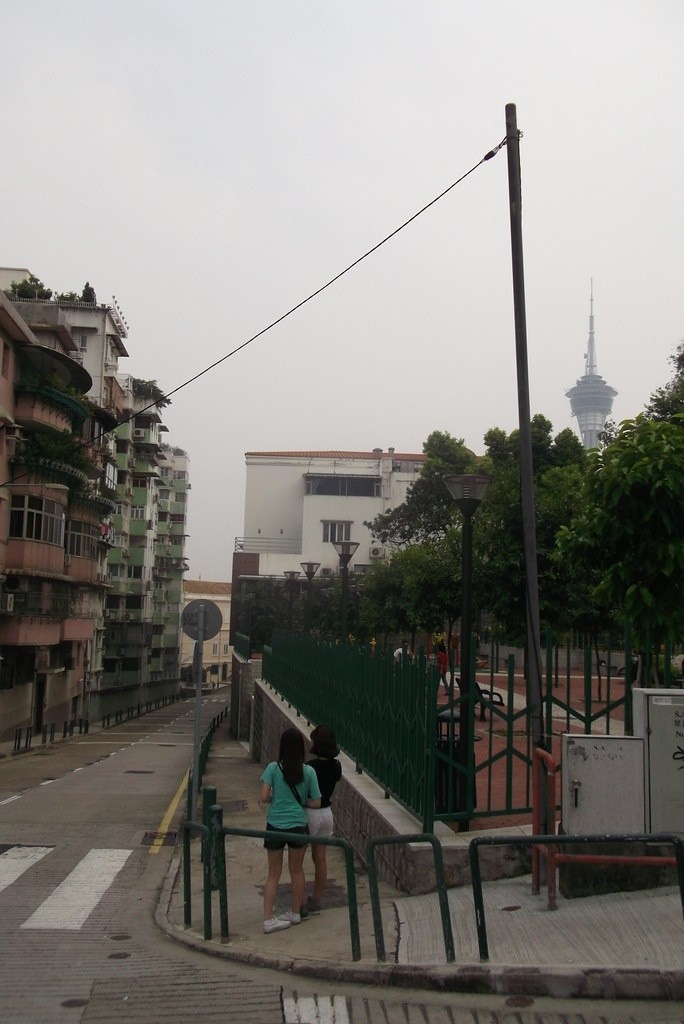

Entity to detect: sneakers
[264,914,292,933]
[277,909,301,924]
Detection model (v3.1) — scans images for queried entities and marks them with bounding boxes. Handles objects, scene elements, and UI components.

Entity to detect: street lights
[300,562,321,635]
[331,541,361,645]
[284,571,301,629]
[442,474,494,831]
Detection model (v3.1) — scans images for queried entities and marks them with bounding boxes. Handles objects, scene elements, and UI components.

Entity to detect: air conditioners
[64,553,72,566]
[321,568,332,576]
[99,457,176,620]
[1,593,15,611]
[370,548,386,558]
[134,428,145,437]
[3,575,30,593]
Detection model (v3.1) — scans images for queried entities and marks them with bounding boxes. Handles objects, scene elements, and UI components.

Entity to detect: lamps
[0,483,69,491]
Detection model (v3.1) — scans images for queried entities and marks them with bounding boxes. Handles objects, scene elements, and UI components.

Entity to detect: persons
[398,641,412,664]
[437,646,449,696]
[299,725,341,920]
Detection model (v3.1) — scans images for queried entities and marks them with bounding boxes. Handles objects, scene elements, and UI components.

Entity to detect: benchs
[456,677,505,720]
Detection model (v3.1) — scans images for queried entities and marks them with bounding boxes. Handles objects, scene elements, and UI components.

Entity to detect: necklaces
[260,727,322,934]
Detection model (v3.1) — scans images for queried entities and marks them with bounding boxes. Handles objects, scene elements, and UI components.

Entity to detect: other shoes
[305,897,320,915]
[299,905,310,920]
